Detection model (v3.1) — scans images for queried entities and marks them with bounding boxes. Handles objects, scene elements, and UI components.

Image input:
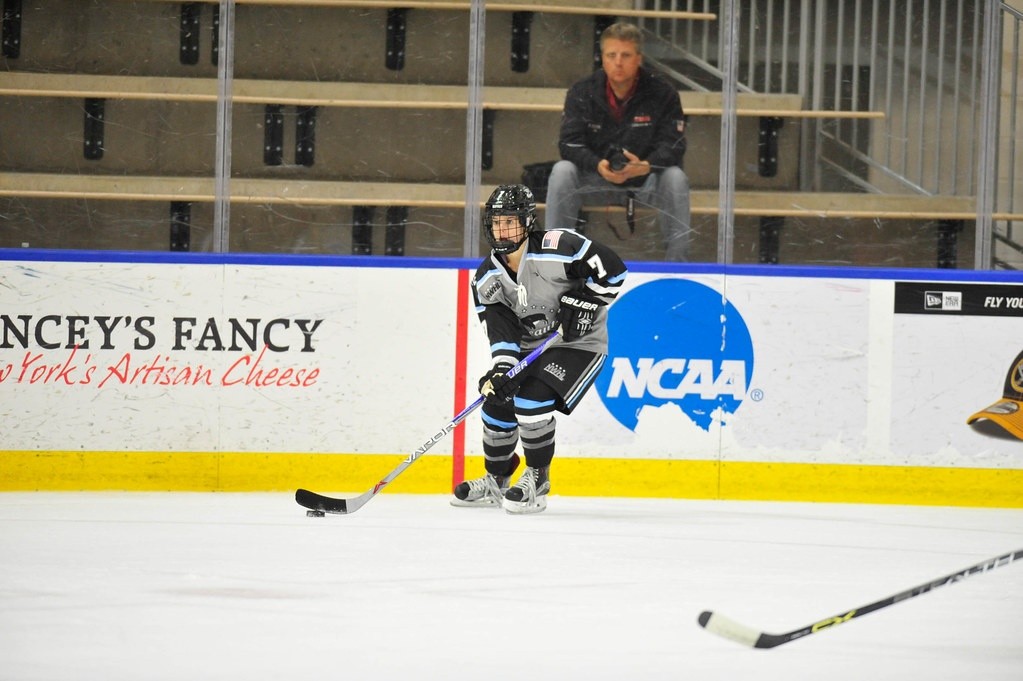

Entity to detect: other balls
[307,510,326,517]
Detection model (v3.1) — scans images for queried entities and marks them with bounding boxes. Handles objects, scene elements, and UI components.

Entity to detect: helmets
[483,184,537,254]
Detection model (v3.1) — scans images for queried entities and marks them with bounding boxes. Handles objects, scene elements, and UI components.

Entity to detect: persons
[545,23,690,263]
[449,184,627,514]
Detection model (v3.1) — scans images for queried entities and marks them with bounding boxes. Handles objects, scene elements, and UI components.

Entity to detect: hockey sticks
[697,549,1021,651]
[296,333,562,513]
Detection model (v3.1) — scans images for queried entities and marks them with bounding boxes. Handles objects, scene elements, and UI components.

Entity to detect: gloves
[479,362,523,406]
[556,289,609,342]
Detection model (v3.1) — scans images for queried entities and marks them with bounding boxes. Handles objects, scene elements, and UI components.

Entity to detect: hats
[967,350,1023,440]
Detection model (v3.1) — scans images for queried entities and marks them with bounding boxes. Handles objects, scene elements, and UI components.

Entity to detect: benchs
[0,171,1023,268]
[0,70,885,179]
[1,0,717,74]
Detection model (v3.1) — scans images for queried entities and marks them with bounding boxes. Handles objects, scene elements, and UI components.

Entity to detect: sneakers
[449,452,520,507]
[502,464,550,515]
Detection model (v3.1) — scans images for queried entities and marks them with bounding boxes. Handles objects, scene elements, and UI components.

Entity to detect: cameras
[604,144,632,171]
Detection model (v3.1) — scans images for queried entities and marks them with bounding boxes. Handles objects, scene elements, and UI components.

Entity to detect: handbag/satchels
[522,160,559,203]
